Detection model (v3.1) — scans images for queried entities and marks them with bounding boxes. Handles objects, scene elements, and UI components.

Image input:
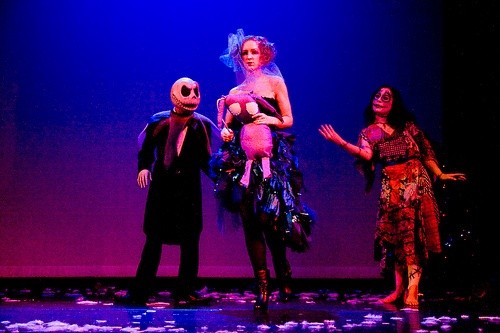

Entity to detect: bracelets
[340,140,346,146]
[437,172,443,177]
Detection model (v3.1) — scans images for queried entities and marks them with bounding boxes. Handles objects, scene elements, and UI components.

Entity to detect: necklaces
[375,121,388,128]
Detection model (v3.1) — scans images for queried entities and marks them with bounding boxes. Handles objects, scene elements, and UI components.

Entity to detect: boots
[251,269,272,313]
[277,271,294,303]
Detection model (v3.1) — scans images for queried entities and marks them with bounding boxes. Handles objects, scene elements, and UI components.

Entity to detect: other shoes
[113,294,147,308]
[174,294,190,310]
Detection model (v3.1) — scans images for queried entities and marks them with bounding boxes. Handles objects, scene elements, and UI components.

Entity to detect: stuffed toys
[217,91,284,186]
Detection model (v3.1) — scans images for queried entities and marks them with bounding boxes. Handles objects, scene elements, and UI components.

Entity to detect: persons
[209,35,317,309]
[317,85,466,305]
[115,77,220,307]
